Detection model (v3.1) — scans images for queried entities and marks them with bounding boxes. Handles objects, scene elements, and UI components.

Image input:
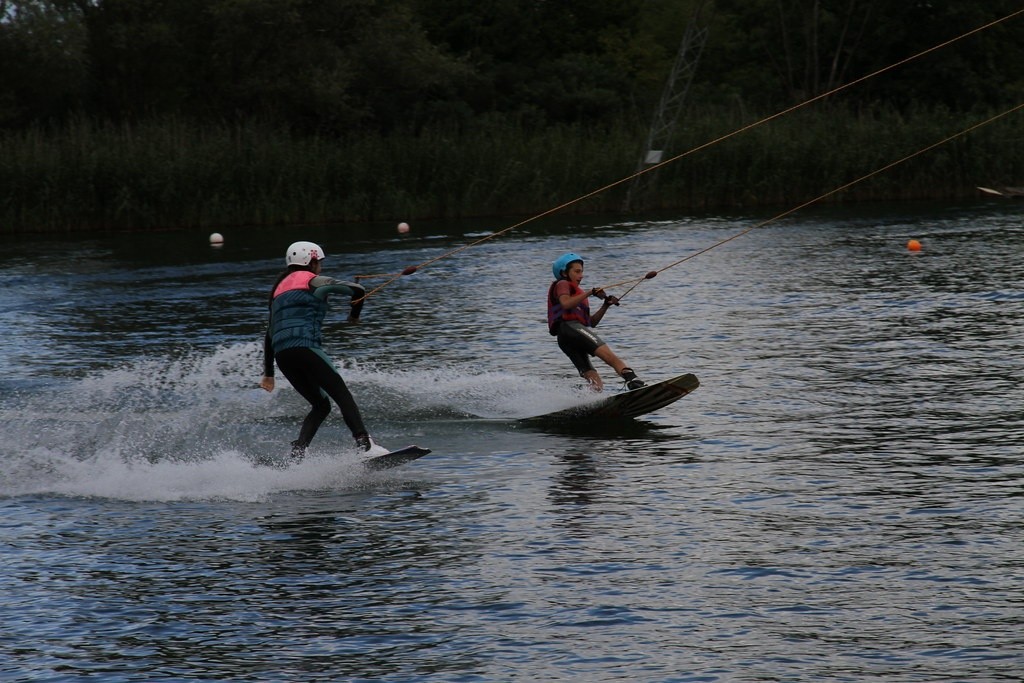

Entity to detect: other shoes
[357,433,390,461]
[617,368,649,392]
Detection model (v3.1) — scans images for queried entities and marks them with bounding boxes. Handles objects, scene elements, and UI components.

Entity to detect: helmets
[553,253,584,280]
[286,241,325,266]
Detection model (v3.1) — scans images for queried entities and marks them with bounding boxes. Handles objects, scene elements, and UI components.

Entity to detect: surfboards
[518,372,701,427]
[362,445,434,473]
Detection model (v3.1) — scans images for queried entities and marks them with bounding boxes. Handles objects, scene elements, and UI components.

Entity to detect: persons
[548,253,648,393]
[262,241,390,461]
